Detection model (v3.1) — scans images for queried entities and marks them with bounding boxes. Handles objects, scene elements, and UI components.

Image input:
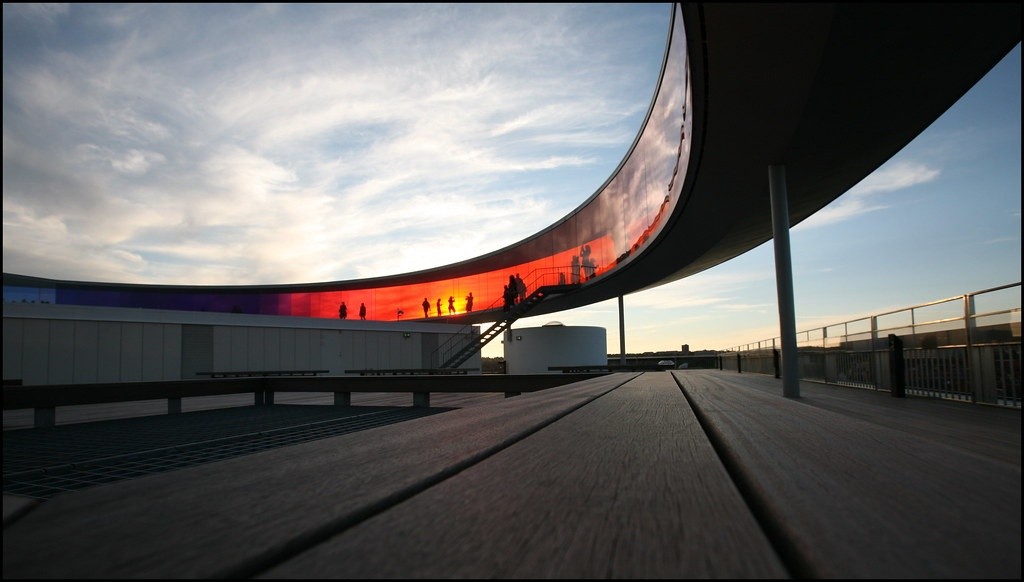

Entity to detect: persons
[339,302,347,320]
[448,296,456,315]
[359,303,366,321]
[466,292,474,312]
[422,298,430,317]
[437,299,442,317]
[502,273,527,306]
[560,245,595,284]
